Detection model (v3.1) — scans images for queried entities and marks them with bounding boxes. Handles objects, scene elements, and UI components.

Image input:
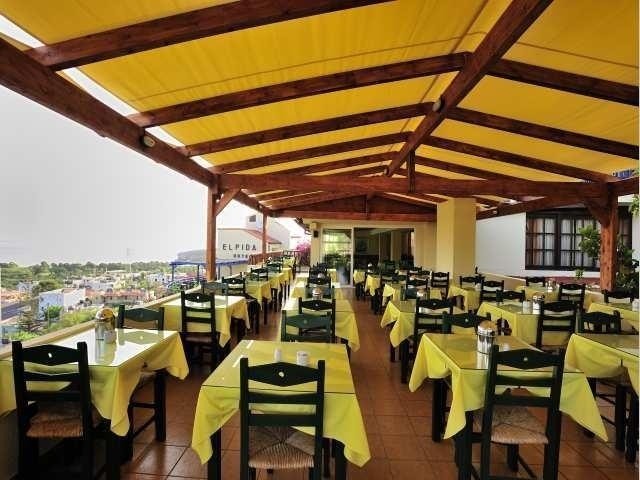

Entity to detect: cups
[297,351,308,367]
[104,330,116,344]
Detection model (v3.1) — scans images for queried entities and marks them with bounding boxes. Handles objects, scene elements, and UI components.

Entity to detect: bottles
[274,348,282,363]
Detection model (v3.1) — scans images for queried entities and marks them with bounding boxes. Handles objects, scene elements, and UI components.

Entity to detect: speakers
[314,231,318,237]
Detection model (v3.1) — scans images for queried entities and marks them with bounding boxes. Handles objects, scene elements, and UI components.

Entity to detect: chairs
[112,303,166,462]
[239,357,331,480]
[454,343,565,479]
[11,340,121,479]
[160,255,639,385]
[577,308,627,451]
